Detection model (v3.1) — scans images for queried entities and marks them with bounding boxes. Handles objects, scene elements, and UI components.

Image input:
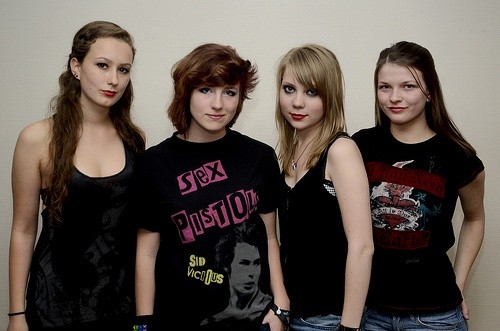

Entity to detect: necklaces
[289,133,316,169]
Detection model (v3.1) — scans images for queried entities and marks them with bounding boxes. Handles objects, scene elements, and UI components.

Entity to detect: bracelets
[270,303,291,329]
[133,314,153,331]
[335,323,361,331]
[7,311,25,317]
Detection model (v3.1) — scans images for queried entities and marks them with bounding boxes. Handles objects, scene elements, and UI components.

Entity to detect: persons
[129,43,291,331]
[350,41,486,331]
[7,21,146,331]
[273,44,376,331]
